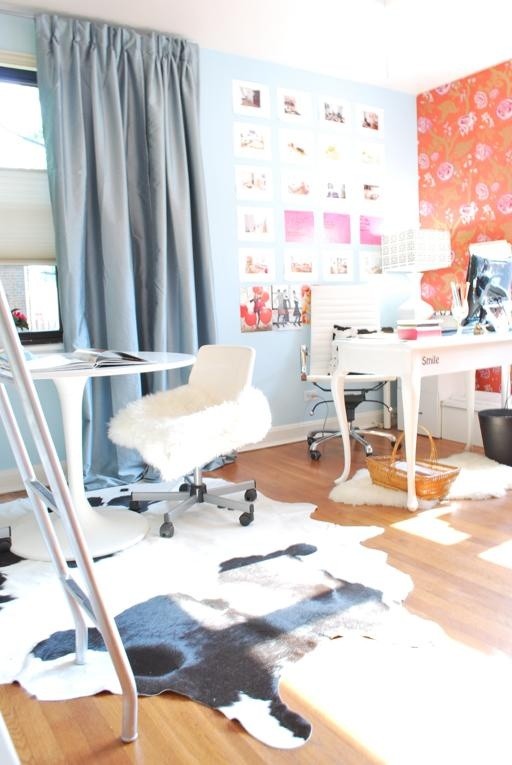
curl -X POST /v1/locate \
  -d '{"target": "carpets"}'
[328,452,511,506]
[0,475,416,751]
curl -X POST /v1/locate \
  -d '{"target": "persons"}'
[249,294,266,326]
[274,288,303,327]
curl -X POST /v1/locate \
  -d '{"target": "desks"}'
[0,351,195,561]
[329,331,512,513]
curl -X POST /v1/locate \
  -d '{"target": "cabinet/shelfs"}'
[396,320,465,438]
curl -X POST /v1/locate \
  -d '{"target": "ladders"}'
[0,278,140,743]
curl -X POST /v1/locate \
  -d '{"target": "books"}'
[27,348,150,372]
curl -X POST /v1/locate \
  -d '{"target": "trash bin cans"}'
[478,409,512,468]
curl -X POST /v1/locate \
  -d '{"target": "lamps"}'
[381,228,453,320]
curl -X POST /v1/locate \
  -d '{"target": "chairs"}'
[105,344,258,536]
[300,283,402,461]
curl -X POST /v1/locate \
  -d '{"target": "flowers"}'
[10,306,30,334]
